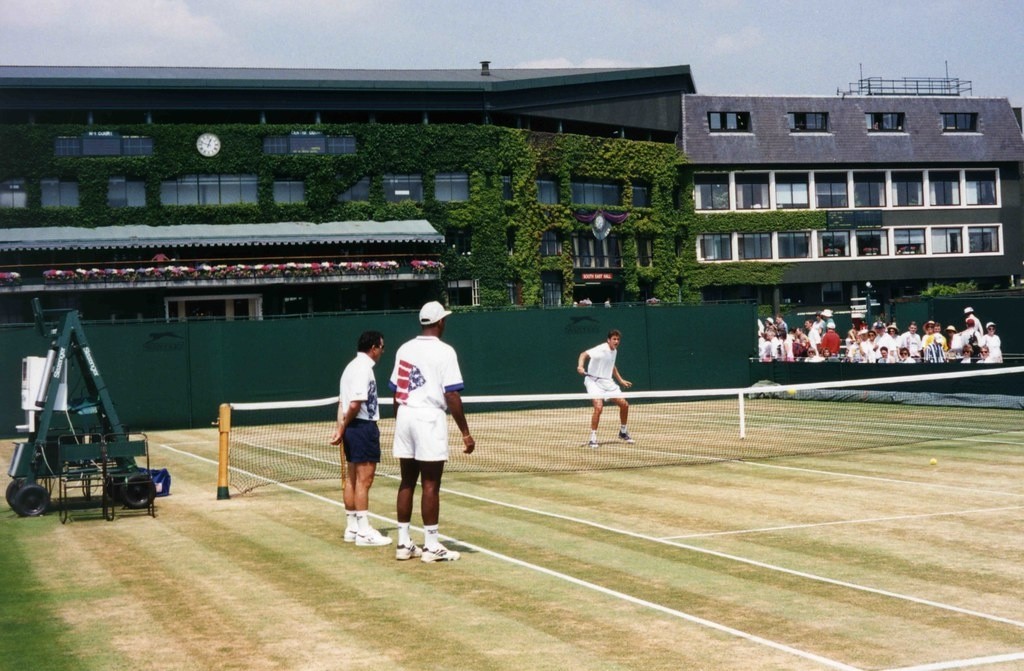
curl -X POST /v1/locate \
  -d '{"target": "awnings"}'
[0,219,446,251]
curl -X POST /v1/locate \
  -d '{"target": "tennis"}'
[788,389,796,394]
[930,458,937,465]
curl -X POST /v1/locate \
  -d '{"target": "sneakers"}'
[421,546,461,564]
[619,430,635,443]
[396,543,422,560]
[589,435,599,448]
[357,529,393,547]
[344,526,358,541]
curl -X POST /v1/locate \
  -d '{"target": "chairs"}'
[54,433,157,524]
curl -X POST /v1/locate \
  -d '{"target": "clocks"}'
[196,132,223,158]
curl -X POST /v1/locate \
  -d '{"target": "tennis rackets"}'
[584,372,618,391]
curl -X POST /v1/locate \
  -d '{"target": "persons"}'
[389,301,475,562]
[576,330,635,448]
[758,307,1003,363]
[329,331,393,547]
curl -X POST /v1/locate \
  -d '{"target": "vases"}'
[901,251,916,254]
[827,254,839,257]
[865,252,878,255]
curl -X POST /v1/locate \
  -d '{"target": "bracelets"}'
[463,435,470,439]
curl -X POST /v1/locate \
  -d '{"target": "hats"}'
[944,325,958,333]
[766,317,774,324]
[820,308,834,317]
[985,322,996,329]
[827,322,836,329]
[964,306,974,314]
[923,321,935,333]
[967,319,975,326]
[419,300,453,325]
[887,324,899,332]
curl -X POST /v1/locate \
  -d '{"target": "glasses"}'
[988,328,995,331]
[374,344,386,350]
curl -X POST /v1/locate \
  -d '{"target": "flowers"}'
[0,259,446,281]
[899,246,917,251]
[862,247,878,253]
[825,248,840,255]
